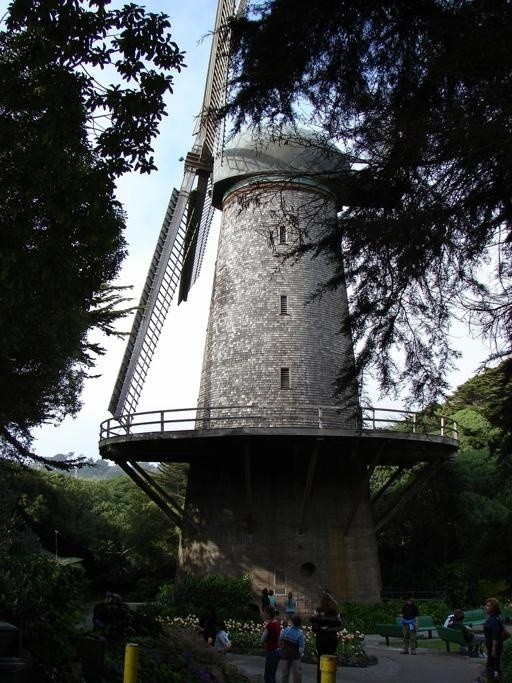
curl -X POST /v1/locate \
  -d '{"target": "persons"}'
[443,609,466,654]
[196,588,343,683]
[447,609,488,658]
[399,596,419,655]
[483,598,504,683]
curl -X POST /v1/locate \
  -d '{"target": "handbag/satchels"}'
[491,617,511,642]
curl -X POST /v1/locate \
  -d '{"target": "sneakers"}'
[399,649,409,654]
[410,648,417,655]
[458,646,488,658]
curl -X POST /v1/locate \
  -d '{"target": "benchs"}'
[396,615,436,638]
[462,608,487,631]
[376,622,417,647]
[435,624,472,653]
